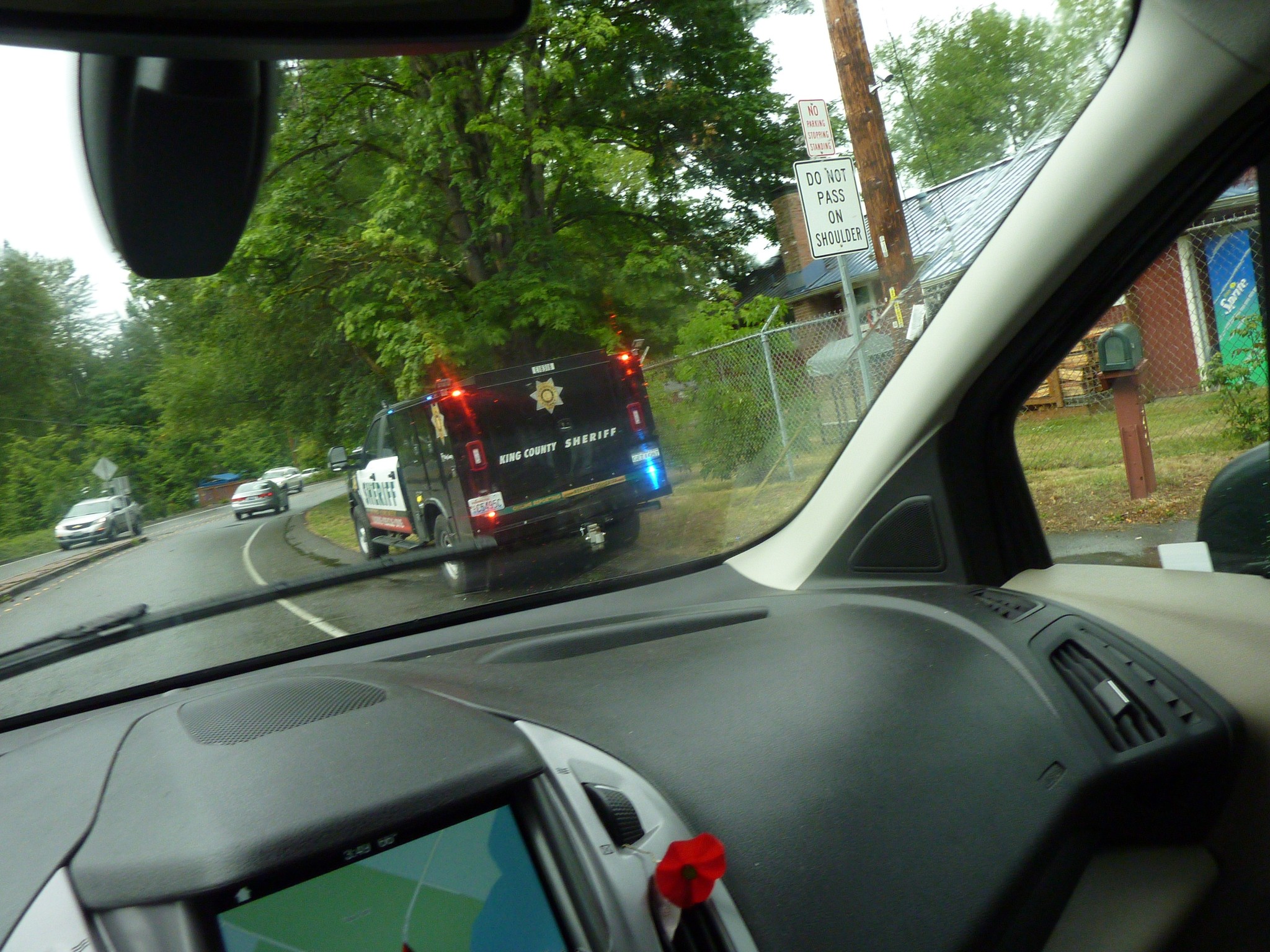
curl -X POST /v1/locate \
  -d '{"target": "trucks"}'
[327,329,675,594]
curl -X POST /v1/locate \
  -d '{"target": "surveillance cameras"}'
[875,68,894,83]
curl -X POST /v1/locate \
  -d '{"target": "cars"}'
[258,467,304,493]
[301,468,319,479]
[54,494,144,550]
[230,479,289,520]
